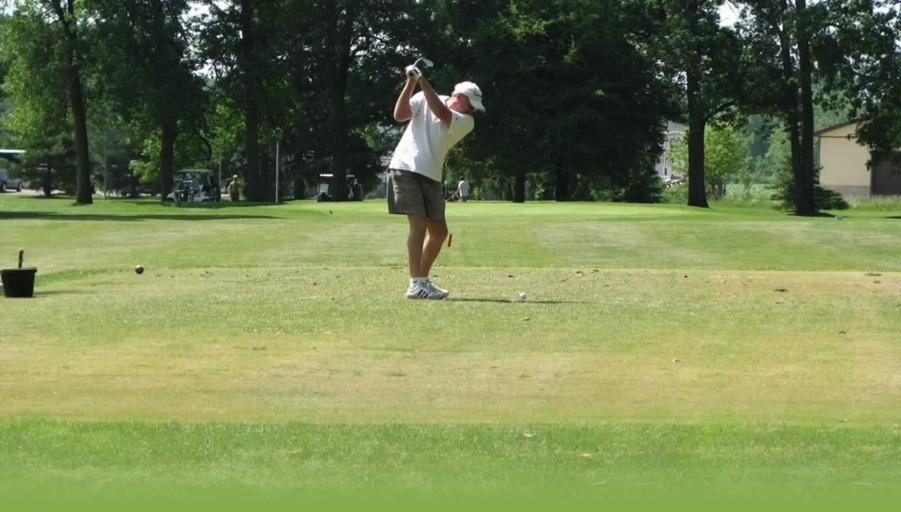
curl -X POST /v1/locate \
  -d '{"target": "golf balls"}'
[135,265,144,273]
[519,292,526,300]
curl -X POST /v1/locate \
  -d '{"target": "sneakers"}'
[425,278,449,300]
[406,280,446,299]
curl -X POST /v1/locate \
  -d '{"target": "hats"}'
[454,81,486,114]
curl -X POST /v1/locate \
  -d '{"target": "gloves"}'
[403,65,421,82]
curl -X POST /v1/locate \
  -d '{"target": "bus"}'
[280,173,355,199]
[0,148,56,192]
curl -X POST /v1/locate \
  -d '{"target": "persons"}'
[348,177,363,200]
[457,176,470,200]
[386,65,486,300]
[169,172,240,201]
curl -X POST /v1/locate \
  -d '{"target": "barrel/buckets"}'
[1,268,37,296]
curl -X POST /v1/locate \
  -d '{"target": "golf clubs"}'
[408,57,433,75]
